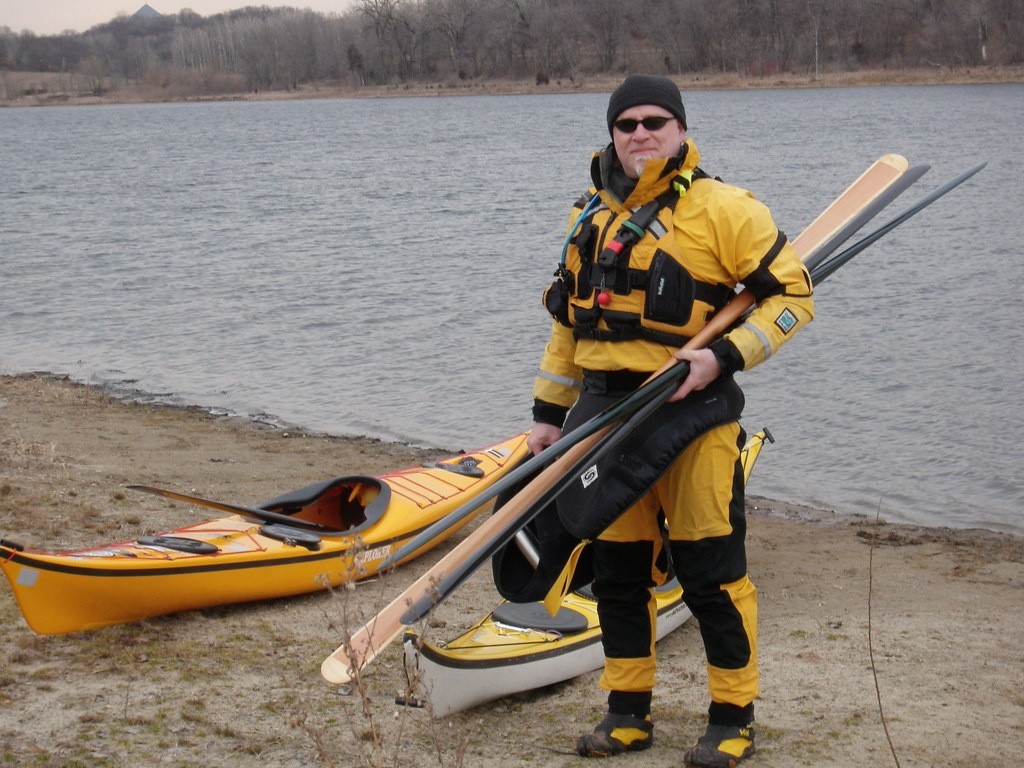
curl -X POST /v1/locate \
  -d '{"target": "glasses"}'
[613,115,679,134]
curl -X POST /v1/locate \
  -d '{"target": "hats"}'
[606,73,688,140]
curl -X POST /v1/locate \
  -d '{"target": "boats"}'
[2,418,541,637]
[402,428,779,723]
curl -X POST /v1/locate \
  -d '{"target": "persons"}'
[529,73,815,768]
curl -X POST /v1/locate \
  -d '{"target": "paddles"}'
[318,152,988,685]
[124,480,342,532]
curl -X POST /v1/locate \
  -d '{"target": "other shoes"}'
[576,712,654,758]
[683,713,756,767]
